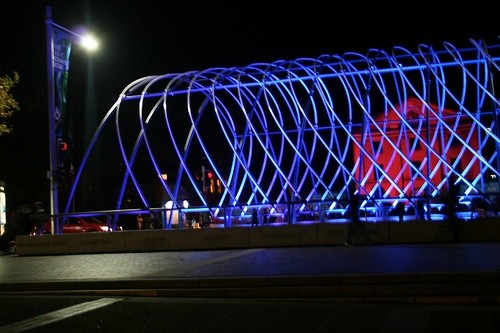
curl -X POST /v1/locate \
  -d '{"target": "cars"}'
[31,216,123,235]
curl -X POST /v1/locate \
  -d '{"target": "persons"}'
[135,200,426,229]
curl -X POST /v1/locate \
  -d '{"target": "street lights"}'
[45,18,96,239]
